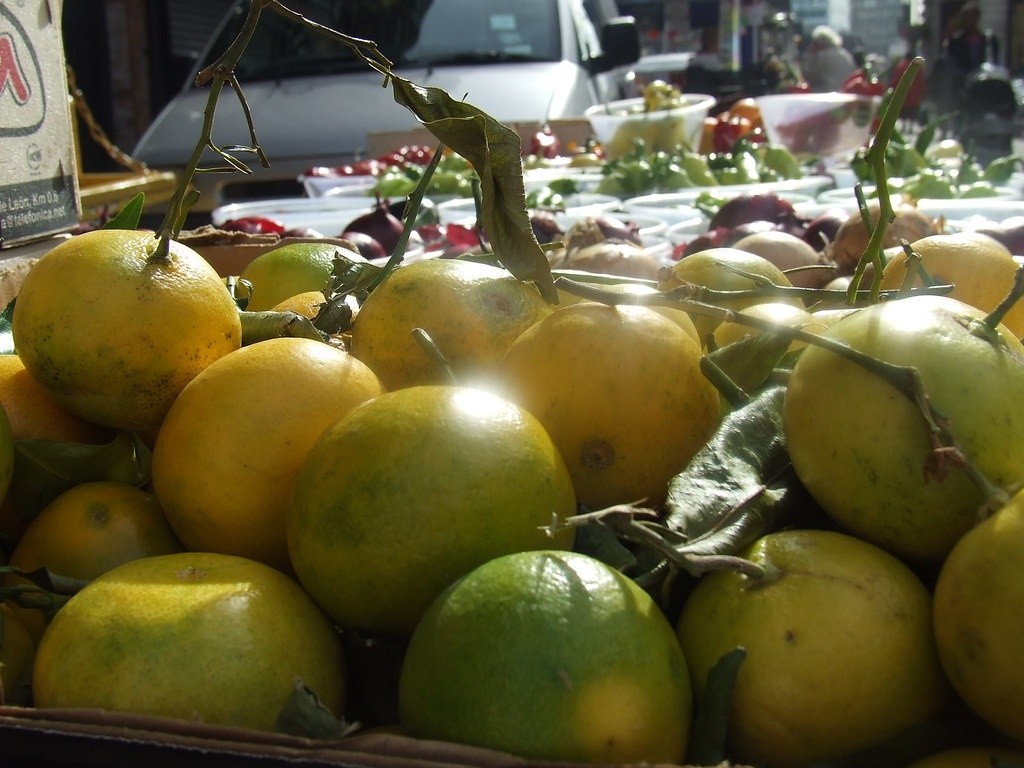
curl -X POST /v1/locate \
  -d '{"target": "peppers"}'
[301,55,1024,203]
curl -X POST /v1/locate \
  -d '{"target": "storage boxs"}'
[370,119,586,170]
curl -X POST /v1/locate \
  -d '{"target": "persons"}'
[683,25,720,98]
[853,50,923,120]
[966,62,1015,166]
[947,1,985,68]
[805,25,857,93]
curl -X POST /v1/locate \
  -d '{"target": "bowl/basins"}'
[583,93,717,159]
[755,92,883,159]
[209,152,1024,236]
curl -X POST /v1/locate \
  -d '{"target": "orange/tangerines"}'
[0,234,1024,768]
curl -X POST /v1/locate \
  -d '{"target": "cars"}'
[129,0,644,179]
